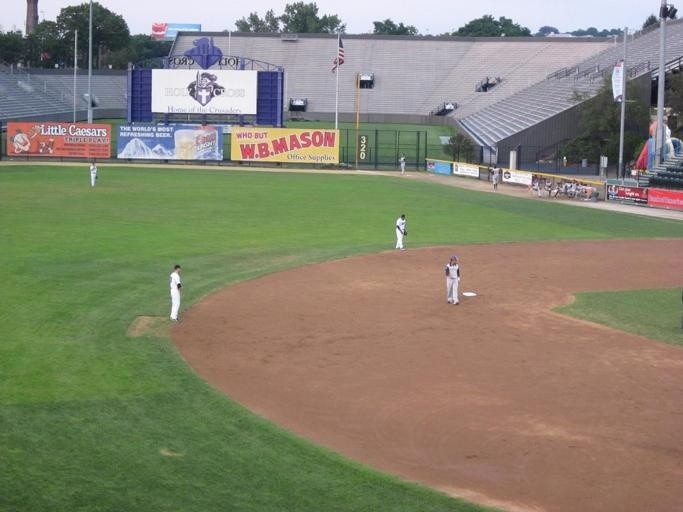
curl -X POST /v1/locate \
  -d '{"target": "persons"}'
[444,255,461,305]
[398,151,406,174]
[491,164,503,192]
[90,161,98,187]
[528,175,597,201]
[169,264,183,321]
[394,213,408,251]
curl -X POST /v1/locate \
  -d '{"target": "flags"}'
[610,59,626,101]
[329,40,345,74]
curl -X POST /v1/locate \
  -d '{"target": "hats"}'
[174,265,184,269]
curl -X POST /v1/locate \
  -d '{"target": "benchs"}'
[0,21,682,192]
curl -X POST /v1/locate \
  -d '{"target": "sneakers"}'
[400,248,406,251]
[447,298,460,305]
[169,317,181,321]
